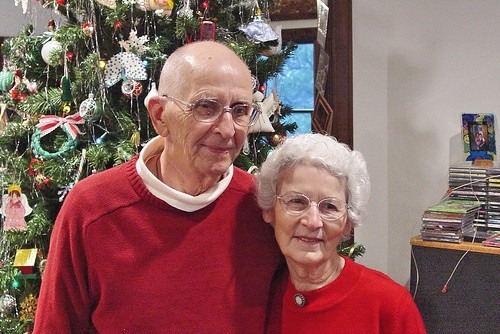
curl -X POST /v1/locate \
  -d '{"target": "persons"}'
[255,134,425,334]
[32,40,279,334]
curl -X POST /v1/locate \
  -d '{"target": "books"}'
[420,113,500,245]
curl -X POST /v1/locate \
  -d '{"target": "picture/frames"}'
[462,113,497,160]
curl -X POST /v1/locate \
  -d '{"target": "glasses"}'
[162,95,263,127]
[275,191,351,222]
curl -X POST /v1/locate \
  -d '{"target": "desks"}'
[410,235,500,334]
